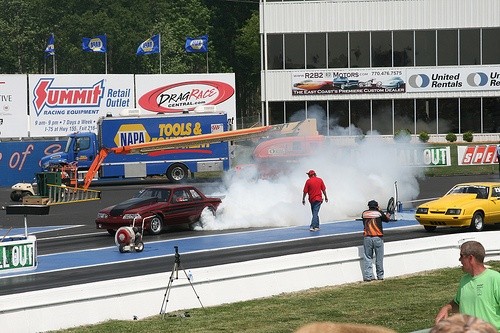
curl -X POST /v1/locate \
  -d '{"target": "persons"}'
[296,320,394,333]
[434,240,500,333]
[428,312,497,333]
[302,170,328,231]
[362,200,390,282]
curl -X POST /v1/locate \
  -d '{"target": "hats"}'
[306,170,315,174]
[368,200,378,206]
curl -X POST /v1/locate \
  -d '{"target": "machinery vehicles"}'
[9,122,281,203]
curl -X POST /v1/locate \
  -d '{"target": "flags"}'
[82,36,107,52]
[136,35,160,55]
[185,35,208,52]
[45,36,55,57]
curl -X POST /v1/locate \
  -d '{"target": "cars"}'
[292,75,384,95]
[95,183,222,237]
[415,182,500,233]
[380,75,406,91]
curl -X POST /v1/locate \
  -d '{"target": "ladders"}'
[46,183,101,205]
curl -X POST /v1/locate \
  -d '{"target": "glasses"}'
[460,253,472,258]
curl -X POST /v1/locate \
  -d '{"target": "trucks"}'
[37,111,234,185]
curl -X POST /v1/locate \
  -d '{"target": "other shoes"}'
[315,228,320,230]
[310,228,314,231]
